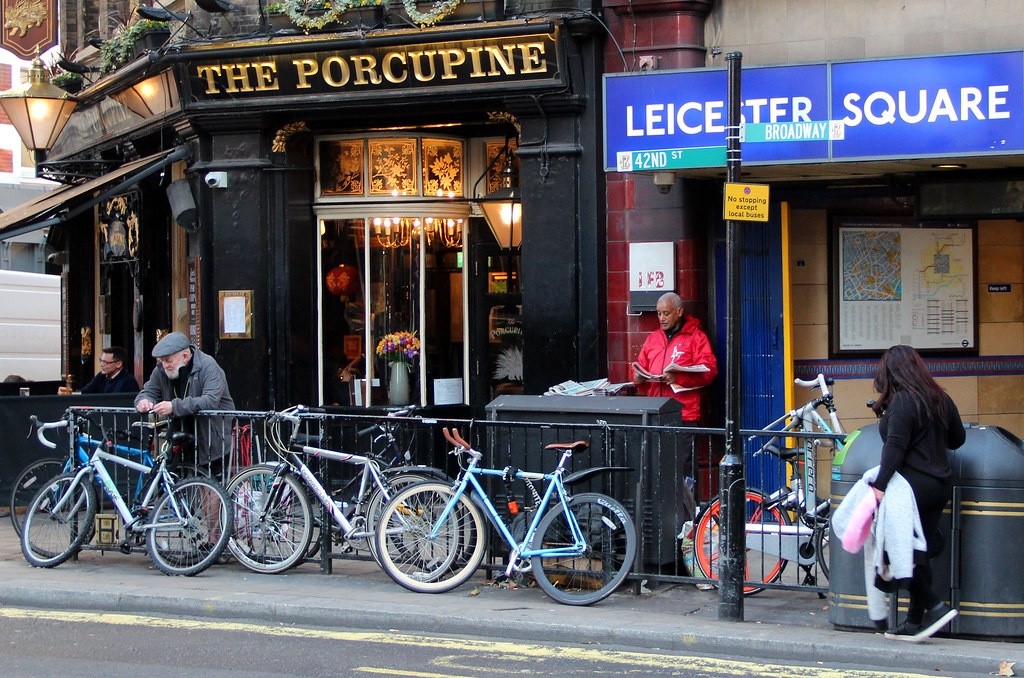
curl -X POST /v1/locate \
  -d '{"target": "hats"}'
[152,332,189,358]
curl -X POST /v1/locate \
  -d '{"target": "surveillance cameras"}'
[47,252,58,265]
[653,172,675,194]
[204,173,220,187]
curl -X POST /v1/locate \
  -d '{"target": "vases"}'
[269,5,380,34]
[391,363,408,405]
[387,0,504,26]
[122,28,171,64]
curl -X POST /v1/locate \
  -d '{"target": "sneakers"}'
[884,620,921,641]
[914,602,958,640]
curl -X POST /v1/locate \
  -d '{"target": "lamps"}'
[0,44,112,185]
[108,68,179,118]
[86,38,105,48]
[374,189,463,249]
[465,125,522,256]
[56,59,105,76]
[195,0,231,13]
[136,7,192,22]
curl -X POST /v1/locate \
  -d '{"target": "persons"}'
[133,333,236,560]
[873,345,965,641]
[632,292,717,506]
[323,353,365,405]
[4,375,25,382]
[78,347,140,392]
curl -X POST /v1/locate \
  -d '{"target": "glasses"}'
[99,358,118,365]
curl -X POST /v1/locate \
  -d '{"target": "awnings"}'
[0,145,191,241]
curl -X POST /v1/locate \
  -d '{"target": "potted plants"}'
[52,73,83,93]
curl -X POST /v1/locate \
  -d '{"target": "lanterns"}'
[326,265,360,302]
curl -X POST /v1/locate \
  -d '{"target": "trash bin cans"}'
[826,423,1024,644]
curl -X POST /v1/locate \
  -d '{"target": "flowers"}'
[260,0,461,30]
[98,20,170,73]
[376,330,420,371]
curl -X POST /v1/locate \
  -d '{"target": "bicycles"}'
[371,425,639,609]
[694,373,884,601]
[7,403,476,584]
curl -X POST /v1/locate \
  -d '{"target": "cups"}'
[20,387,30,397]
